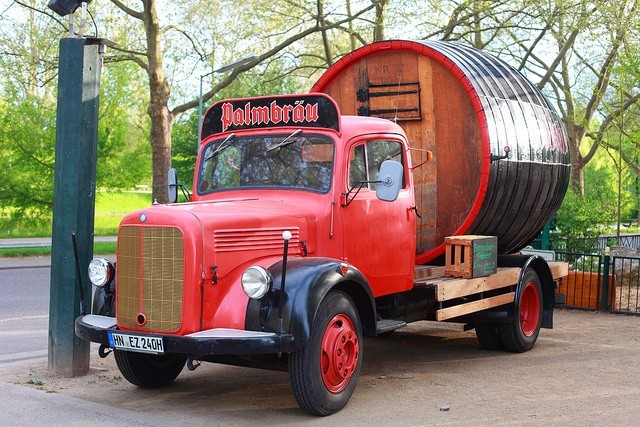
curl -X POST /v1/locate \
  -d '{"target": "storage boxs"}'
[444,235,498,277]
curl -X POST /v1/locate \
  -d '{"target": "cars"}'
[71,39,571,417]
